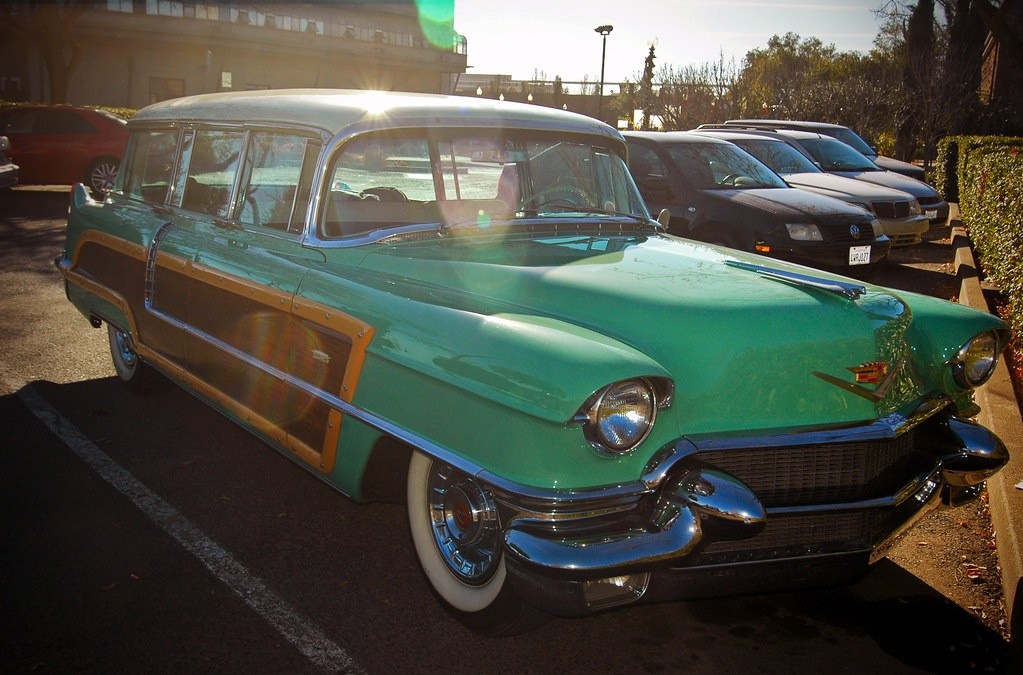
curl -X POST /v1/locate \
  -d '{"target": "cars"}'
[724,117,932,186]
[492,132,889,282]
[0,102,175,201]
[57,85,1013,635]
[750,131,953,241]
[690,123,929,251]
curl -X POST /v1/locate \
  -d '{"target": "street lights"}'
[594,24,614,121]
